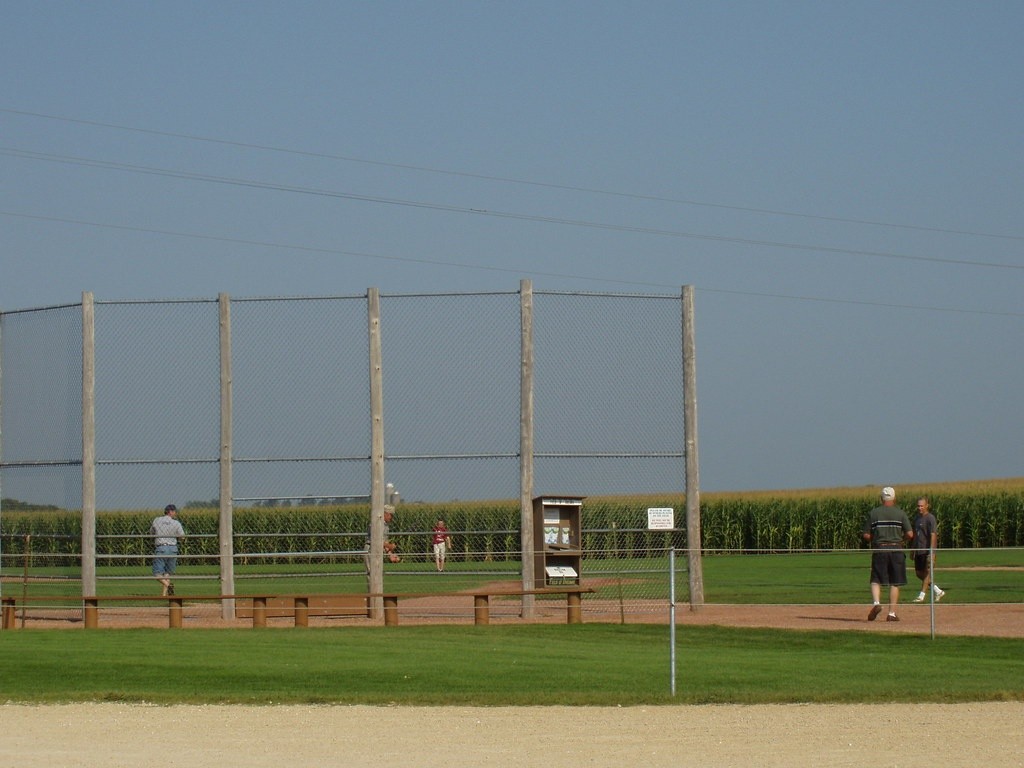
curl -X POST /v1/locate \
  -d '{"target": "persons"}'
[910,496,946,603]
[149,504,185,596]
[363,504,400,582]
[863,486,913,621]
[431,519,451,572]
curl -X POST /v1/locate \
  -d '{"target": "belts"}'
[881,543,901,546]
[157,544,171,546]
[366,542,370,545]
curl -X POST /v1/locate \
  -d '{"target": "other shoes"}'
[437,569,440,572]
[168,583,175,596]
[868,604,881,621]
[934,590,946,602]
[441,569,443,572]
[911,597,925,604]
[887,612,900,620]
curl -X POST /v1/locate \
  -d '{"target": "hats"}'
[165,505,176,510]
[881,487,895,501]
[384,504,395,513]
